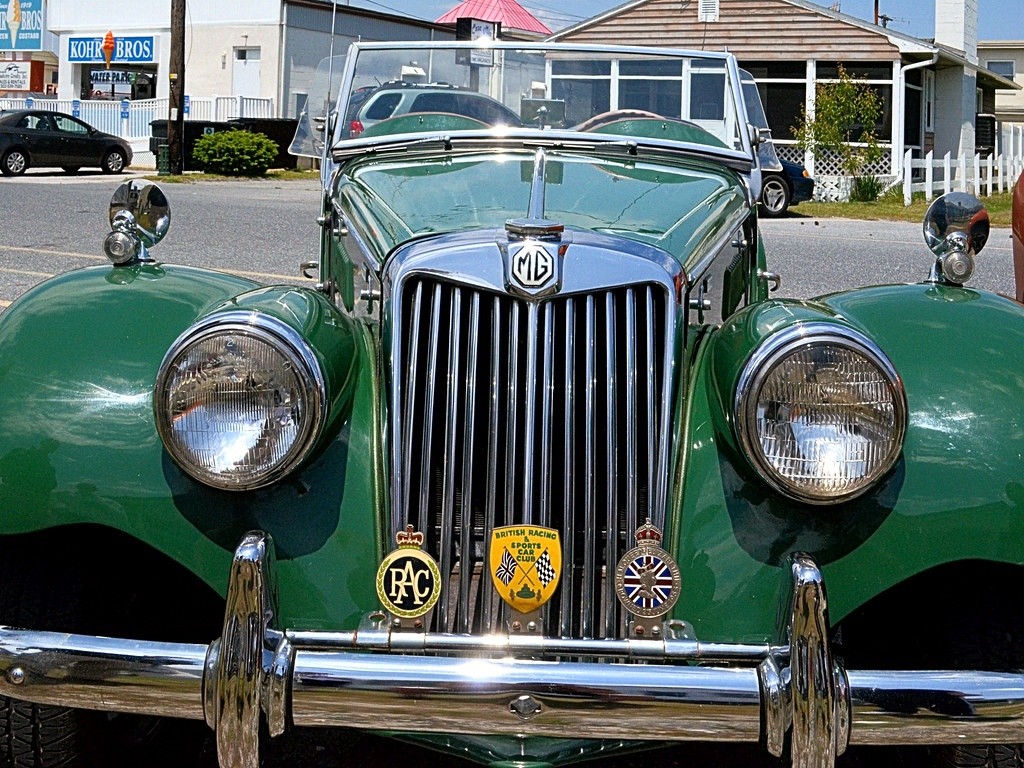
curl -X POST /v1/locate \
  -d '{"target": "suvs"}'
[322,79,528,135]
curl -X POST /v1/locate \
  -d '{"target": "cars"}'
[757,148,815,218]
[0,108,134,176]
[1012,169,1023,304]
[0,42,1023,767]
